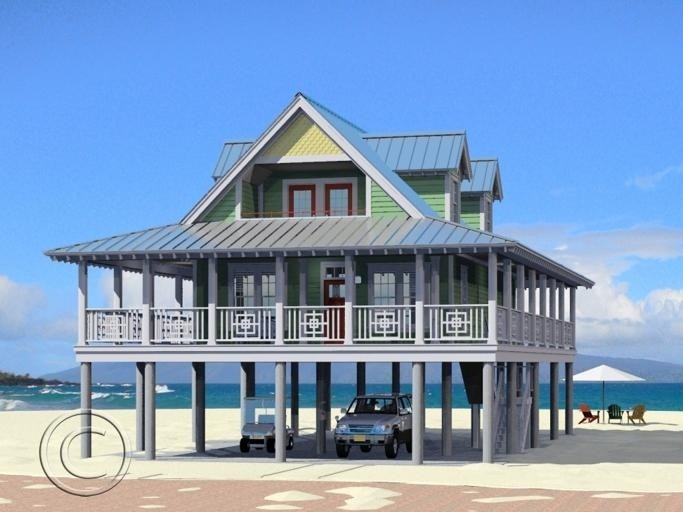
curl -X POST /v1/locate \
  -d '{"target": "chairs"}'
[577,403,646,425]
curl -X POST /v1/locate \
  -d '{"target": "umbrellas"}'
[559,362,645,422]
